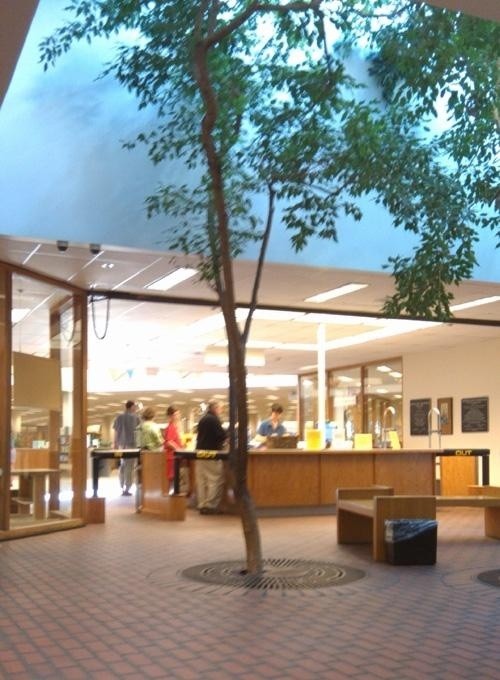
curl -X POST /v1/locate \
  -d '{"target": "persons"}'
[134,407,165,449]
[194,401,228,517]
[161,405,187,492]
[112,399,141,497]
[254,402,288,444]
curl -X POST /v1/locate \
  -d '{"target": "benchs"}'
[336,484,500,566]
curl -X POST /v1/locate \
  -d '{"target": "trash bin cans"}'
[384,518,438,565]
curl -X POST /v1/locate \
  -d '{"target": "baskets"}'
[265,433,300,449]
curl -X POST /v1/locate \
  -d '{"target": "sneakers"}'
[122,491,132,496]
[200,507,223,515]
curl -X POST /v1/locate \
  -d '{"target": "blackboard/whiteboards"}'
[461,397,488,431]
[410,399,431,435]
